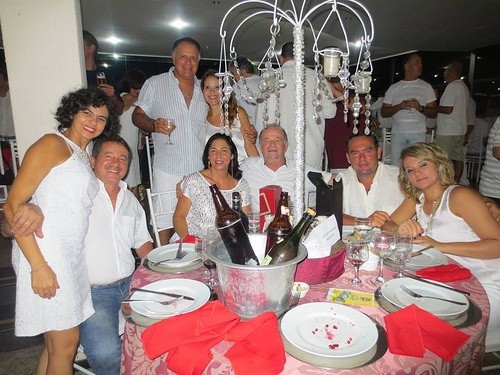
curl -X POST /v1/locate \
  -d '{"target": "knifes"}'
[178,237,183,254]
[411,245,435,257]
[131,287,195,301]
[402,273,470,295]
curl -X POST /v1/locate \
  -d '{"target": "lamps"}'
[214,0,375,227]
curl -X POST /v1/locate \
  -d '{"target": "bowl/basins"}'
[291,282,310,298]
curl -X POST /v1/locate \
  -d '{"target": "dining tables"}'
[121,228,500,375]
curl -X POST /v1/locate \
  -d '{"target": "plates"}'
[146,242,201,265]
[143,259,205,274]
[281,302,379,358]
[121,290,162,327]
[387,243,448,268]
[380,277,469,320]
[128,278,211,320]
[278,315,377,369]
[373,285,468,326]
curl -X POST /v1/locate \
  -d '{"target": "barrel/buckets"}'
[203,233,309,319]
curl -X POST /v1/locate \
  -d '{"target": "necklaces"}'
[425,197,437,204]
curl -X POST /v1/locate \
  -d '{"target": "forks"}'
[119,296,184,306]
[400,285,467,305]
[276,290,301,320]
[154,251,188,266]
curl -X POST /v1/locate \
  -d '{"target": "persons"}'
[83,30,154,203]
[228,41,392,214]
[381,142,500,331]
[462,80,500,209]
[201,69,260,162]
[132,38,257,247]
[176,124,337,232]
[381,54,438,167]
[436,64,469,183]
[0,62,20,185]
[334,131,500,231]
[2,88,121,375]
[1,135,154,375]
[172,133,252,242]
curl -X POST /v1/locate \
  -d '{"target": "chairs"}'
[466,127,485,181]
[381,128,435,167]
[145,189,177,245]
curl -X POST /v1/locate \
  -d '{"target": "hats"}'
[443,61,464,77]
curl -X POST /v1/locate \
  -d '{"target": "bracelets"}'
[420,106,424,113]
[31,263,47,272]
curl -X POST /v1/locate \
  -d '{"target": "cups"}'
[354,216,372,244]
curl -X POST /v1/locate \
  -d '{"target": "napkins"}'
[384,304,472,362]
[141,301,286,375]
[417,264,471,283]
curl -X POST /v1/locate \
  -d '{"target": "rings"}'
[44,291,49,294]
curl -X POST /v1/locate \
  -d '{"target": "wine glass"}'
[393,232,413,279]
[346,240,369,287]
[369,230,397,287]
[163,118,175,145]
[407,96,415,115]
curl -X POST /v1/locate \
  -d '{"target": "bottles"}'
[208,184,258,266]
[230,191,249,234]
[264,190,293,257]
[261,207,317,266]
